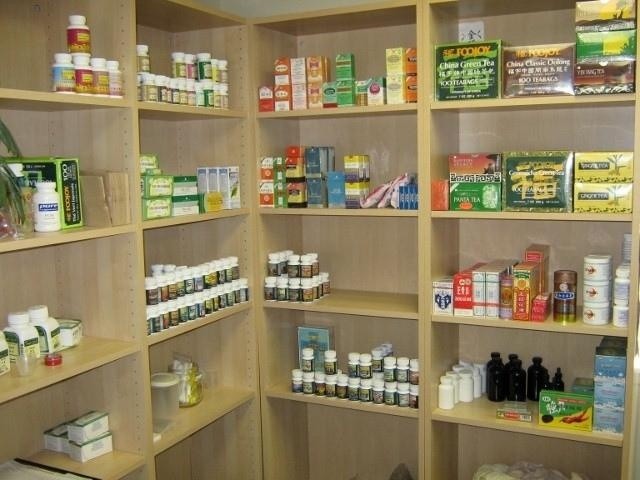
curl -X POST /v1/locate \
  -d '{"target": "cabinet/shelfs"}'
[0,0,264,480]
[248,1,639,480]
